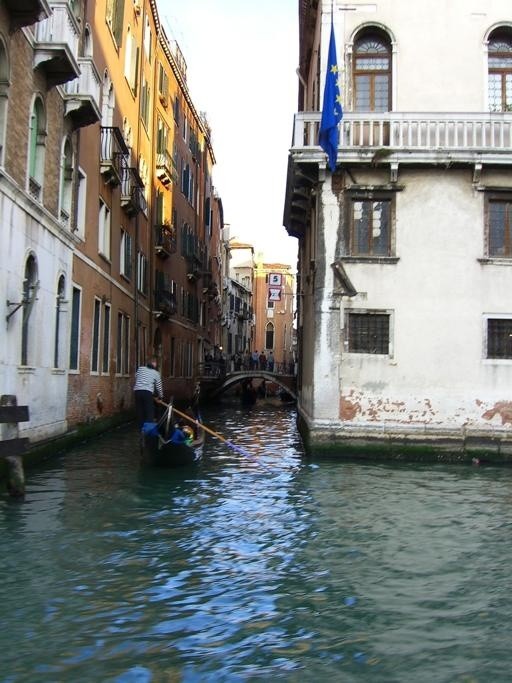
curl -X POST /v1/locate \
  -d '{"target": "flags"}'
[318,20,343,172]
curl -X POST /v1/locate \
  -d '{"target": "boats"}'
[138,404,206,465]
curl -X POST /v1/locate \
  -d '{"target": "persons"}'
[134,361,163,422]
[204,350,274,375]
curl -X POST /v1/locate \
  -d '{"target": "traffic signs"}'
[269,272,282,286]
